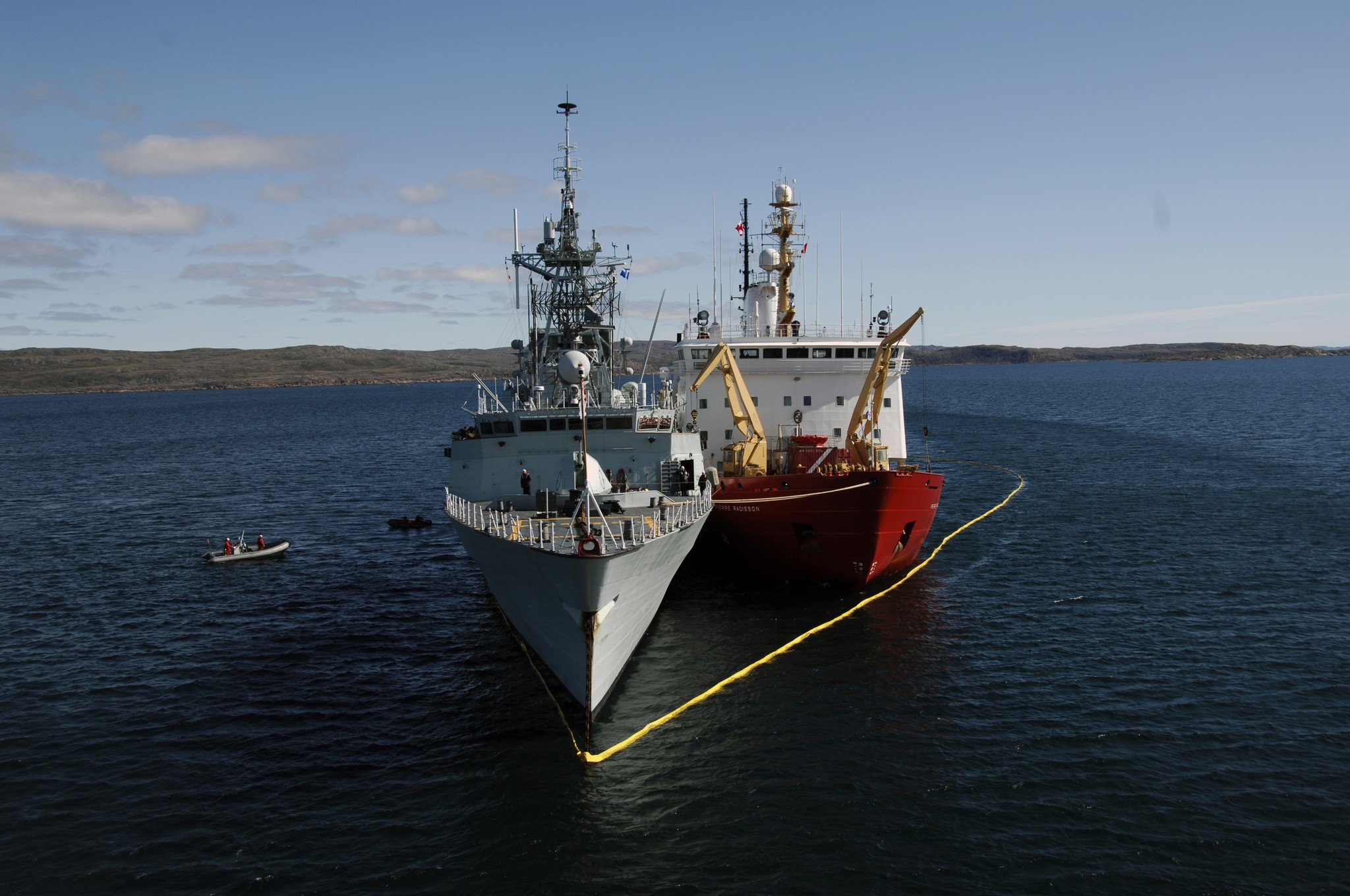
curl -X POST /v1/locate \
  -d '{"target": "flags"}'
[735,219,744,236]
[578,378,587,420]
[620,267,630,280]
[801,243,808,254]
[866,406,873,421]
[509,276,511,282]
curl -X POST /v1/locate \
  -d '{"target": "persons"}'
[225,538,233,555]
[674,466,690,496]
[520,469,532,495]
[401,516,412,524]
[832,466,837,475]
[866,460,872,471]
[414,514,424,524]
[879,464,886,471]
[698,471,708,498]
[257,535,265,550]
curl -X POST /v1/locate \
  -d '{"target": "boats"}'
[790,435,828,445]
[675,166,943,599]
[200,529,291,564]
[387,515,432,529]
[442,91,715,729]
